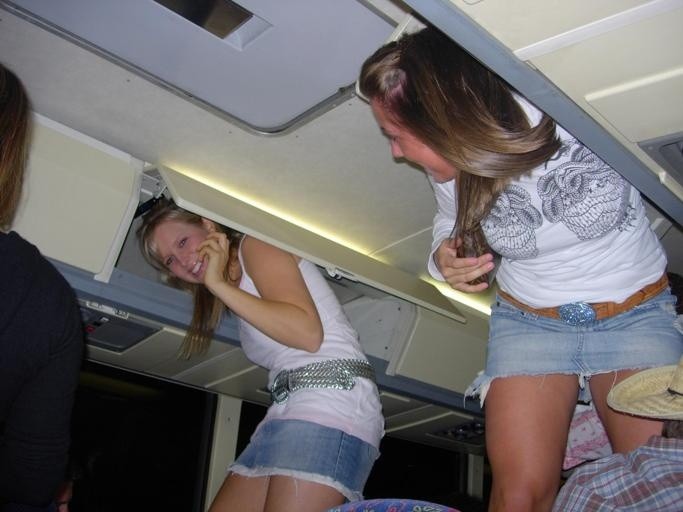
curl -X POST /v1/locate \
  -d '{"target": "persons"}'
[138,199,386,511]
[0,66,86,512]
[359,28,683,512]
[550,355,683,512]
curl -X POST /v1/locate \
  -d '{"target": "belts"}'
[493,271,670,326]
[270,358,377,404]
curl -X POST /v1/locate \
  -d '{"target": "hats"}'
[606,353,682,421]
